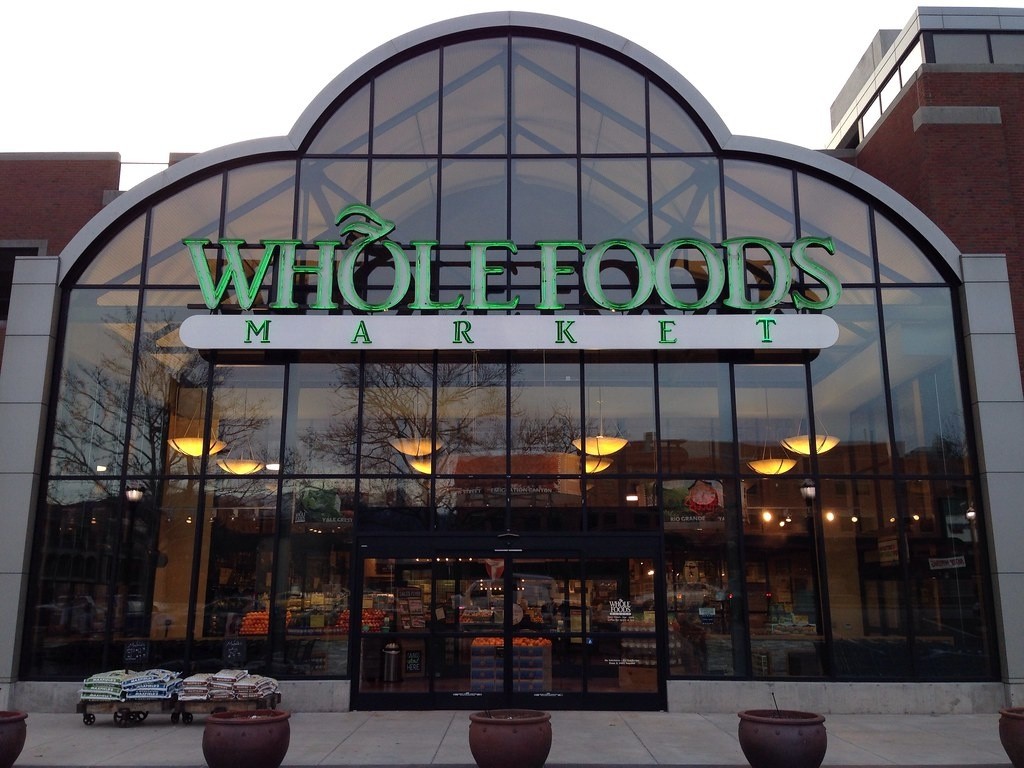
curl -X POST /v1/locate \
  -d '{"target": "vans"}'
[461,579,509,616]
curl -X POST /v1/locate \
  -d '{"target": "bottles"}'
[620,635,683,666]
[557,620,564,641]
[526,608,538,619]
[489,607,496,622]
[620,616,680,632]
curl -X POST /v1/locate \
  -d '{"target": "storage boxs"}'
[471,637,552,694]
[766,604,816,635]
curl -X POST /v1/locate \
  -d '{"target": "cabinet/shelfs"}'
[621,630,682,668]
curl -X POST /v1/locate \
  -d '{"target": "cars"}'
[204,598,267,637]
[633,580,717,612]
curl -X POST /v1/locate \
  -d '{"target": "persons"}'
[541,598,558,629]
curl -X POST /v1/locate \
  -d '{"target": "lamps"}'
[573,387,629,474]
[390,386,444,474]
[167,387,263,473]
[745,386,840,475]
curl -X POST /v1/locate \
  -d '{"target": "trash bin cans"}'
[383,642,401,682]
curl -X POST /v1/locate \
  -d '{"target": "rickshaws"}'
[75,695,283,724]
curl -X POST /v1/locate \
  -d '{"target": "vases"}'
[999,708,1024,768]
[0,710,29,768]
[736,709,828,768]
[469,709,552,768]
[201,712,293,768]
